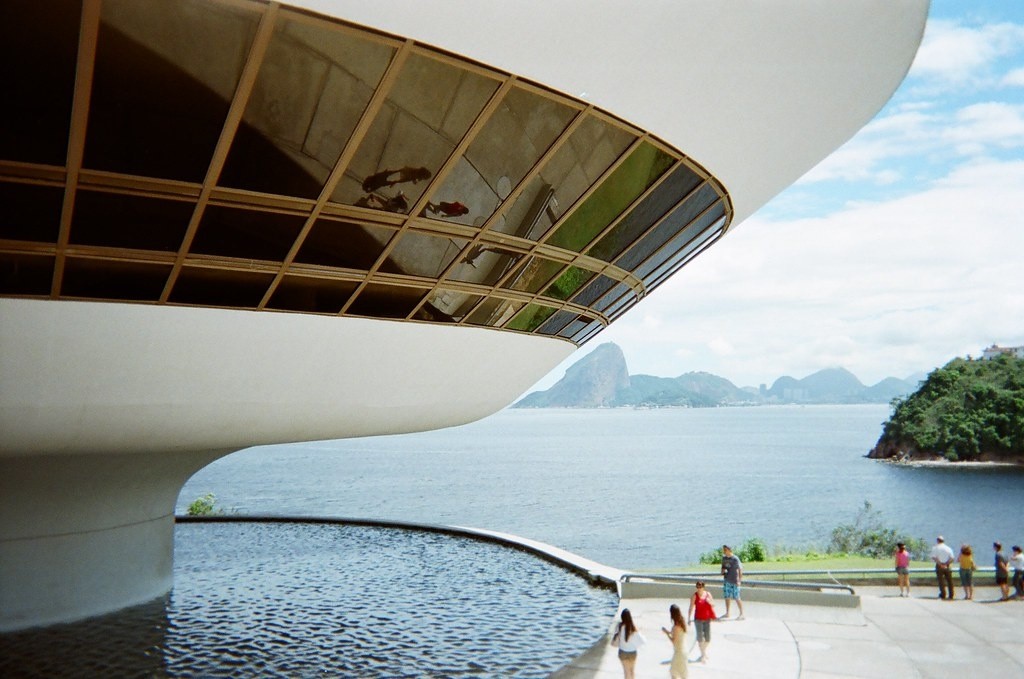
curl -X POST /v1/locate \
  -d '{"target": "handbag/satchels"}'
[710,606,717,620]
[611,623,621,647]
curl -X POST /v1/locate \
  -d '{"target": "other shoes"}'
[900,593,904,596]
[701,655,709,665]
[721,615,729,617]
[907,593,911,597]
[736,616,745,620]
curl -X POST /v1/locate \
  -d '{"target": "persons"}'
[956,545,975,600]
[660,603,690,679]
[992,542,1011,601]
[720,544,746,621]
[354,167,520,266]
[930,536,954,600]
[687,581,715,663]
[1011,545,1024,601]
[611,609,645,679]
[894,541,911,597]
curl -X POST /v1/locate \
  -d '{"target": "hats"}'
[897,541,906,546]
